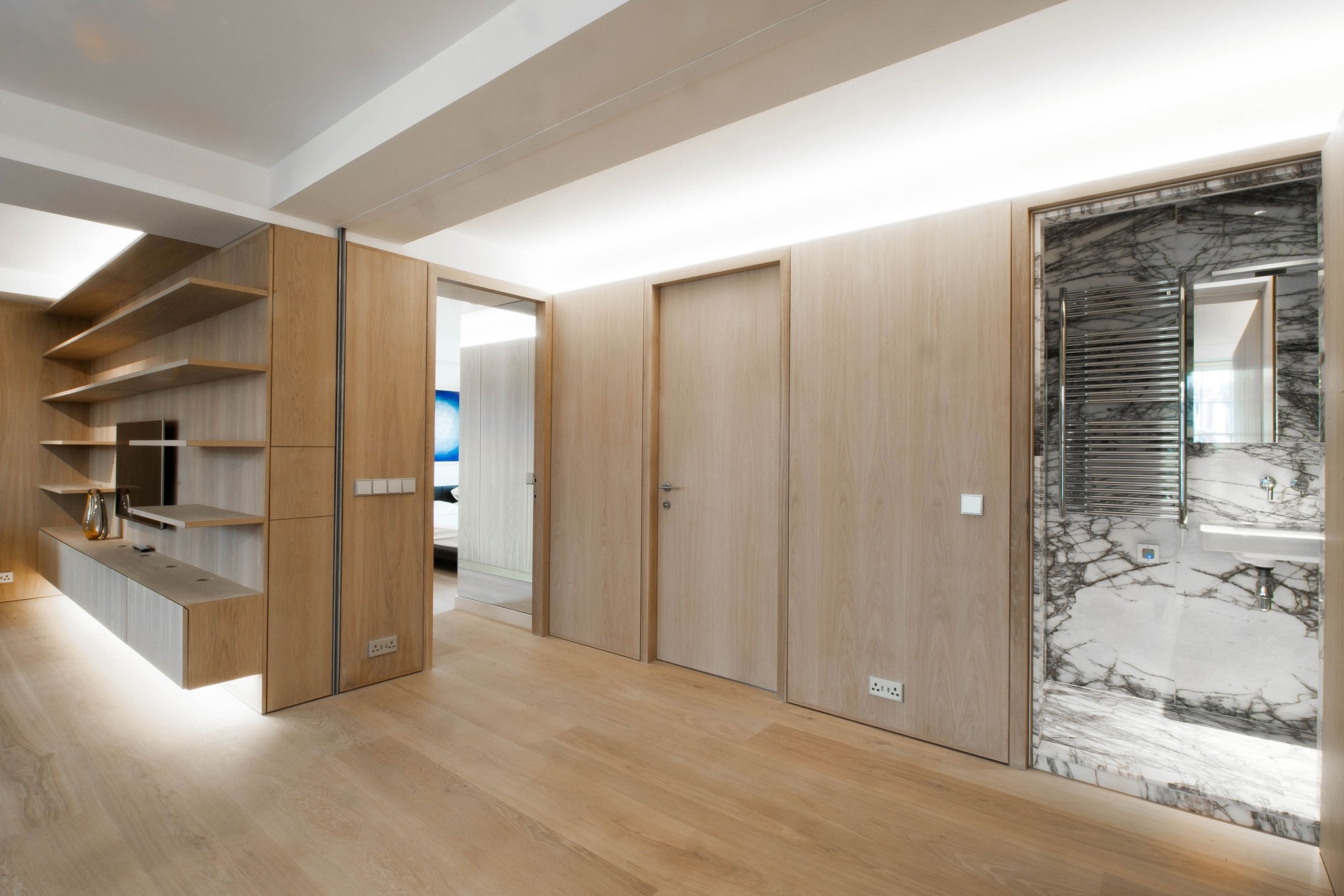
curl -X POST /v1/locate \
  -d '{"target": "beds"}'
[433,484,459,573]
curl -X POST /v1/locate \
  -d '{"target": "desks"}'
[868,675,904,704]
[367,635,398,659]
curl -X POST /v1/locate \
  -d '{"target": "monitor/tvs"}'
[114,419,170,530]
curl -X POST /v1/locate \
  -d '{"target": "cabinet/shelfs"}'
[38,231,266,694]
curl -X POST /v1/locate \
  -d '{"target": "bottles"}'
[116,487,132,519]
[82,488,108,541]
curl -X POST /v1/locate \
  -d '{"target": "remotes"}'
[133,545,151,552]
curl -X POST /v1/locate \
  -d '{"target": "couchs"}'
[451,486,458,502]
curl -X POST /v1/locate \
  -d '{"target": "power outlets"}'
[0,572,13,584]
